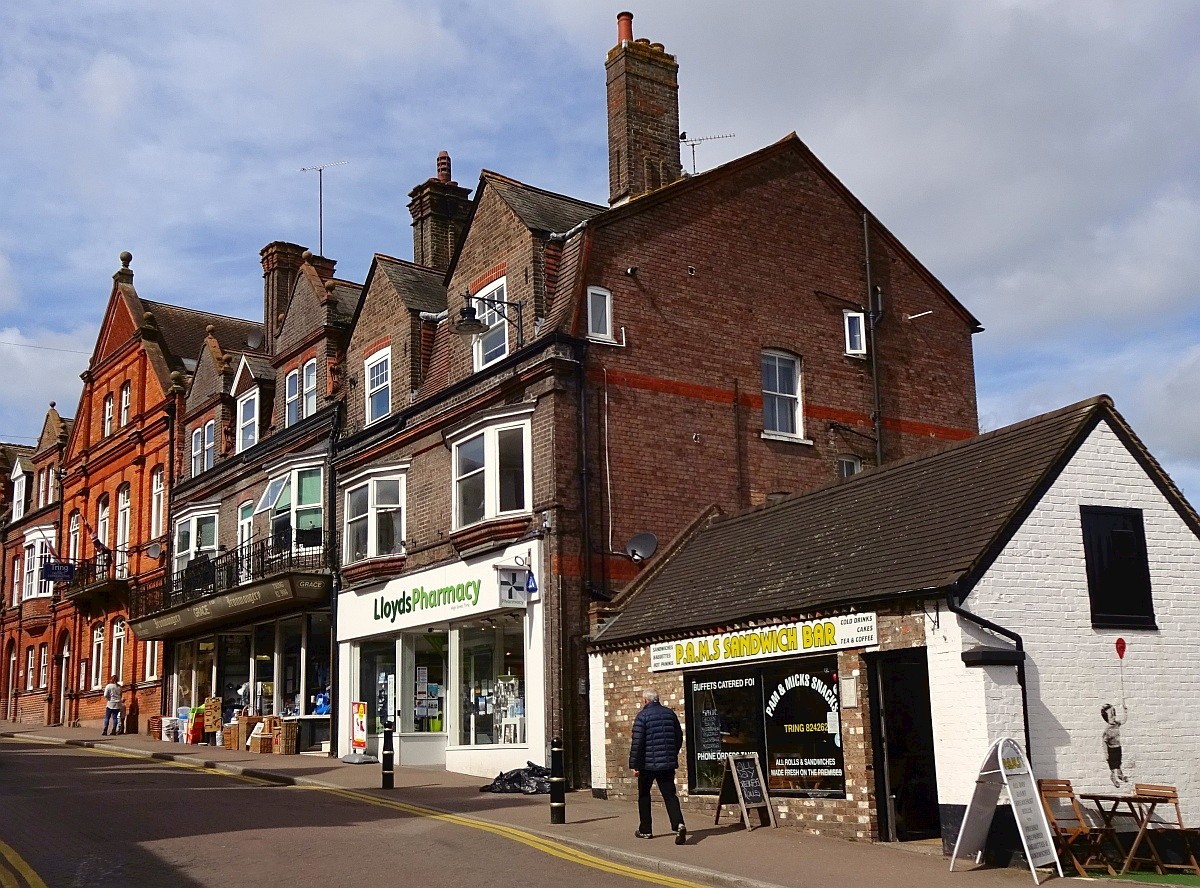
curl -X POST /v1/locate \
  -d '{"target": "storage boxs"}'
[161,696,299,755]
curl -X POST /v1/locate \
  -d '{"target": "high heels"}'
[110,731,117,736]
[102,731,107,736]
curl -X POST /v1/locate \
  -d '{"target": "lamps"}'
[510,612,520,624]
[486,618,497,629]
[448,286,522,348]
[825,421,877,446]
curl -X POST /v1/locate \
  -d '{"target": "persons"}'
[102,675,122,736]
[629,689,687,845]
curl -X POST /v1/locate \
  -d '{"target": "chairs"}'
[1038,779,1117,878]
[1136,784,1200,876]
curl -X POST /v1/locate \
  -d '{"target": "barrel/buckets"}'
[177,707,192,719]
[150,716,162,739]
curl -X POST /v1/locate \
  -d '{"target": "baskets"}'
[239,705,261,724]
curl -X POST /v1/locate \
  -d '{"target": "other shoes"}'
[635,830,652,838]
[117,730,124,735]
[675,825,687,845]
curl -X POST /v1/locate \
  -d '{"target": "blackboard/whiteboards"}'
[699,709,721,754]
[719,754,769,805]
[951,737,1056,866]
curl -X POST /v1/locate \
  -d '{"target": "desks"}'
[502,717,526,744]
[1080,794,1169,876]
